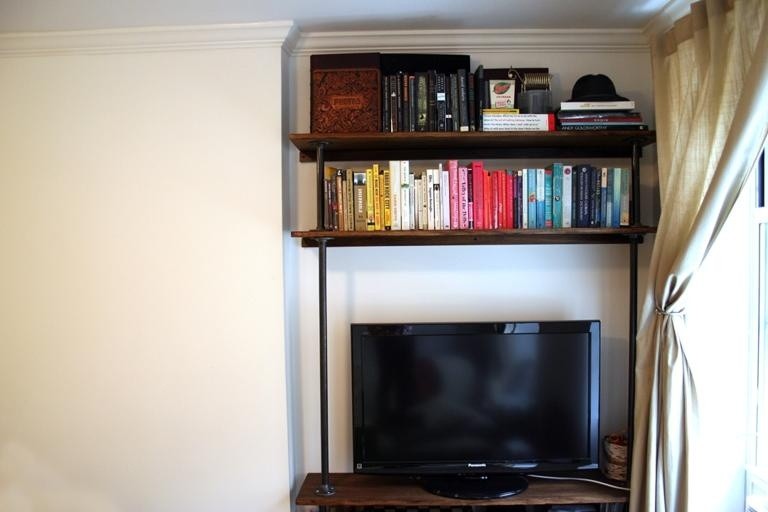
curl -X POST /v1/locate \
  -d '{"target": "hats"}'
[554,74,630,115]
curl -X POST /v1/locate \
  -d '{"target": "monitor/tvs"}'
[350,320,601,500]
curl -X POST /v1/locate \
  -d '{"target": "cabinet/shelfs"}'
[288,131,658,512]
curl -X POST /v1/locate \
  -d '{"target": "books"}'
[323,159,630,231]
[310,53,648,134]
